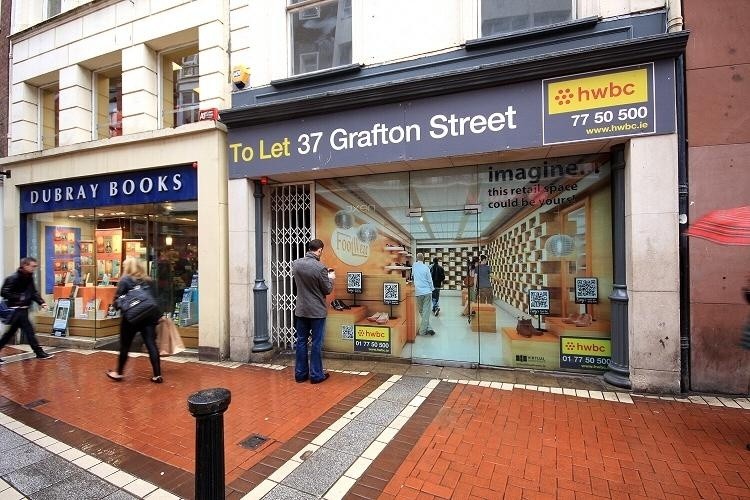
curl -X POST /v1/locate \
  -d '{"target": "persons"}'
[411,252,434,337]
[462,257,478,317]
[0,256,54,365]
[474,254,502,305]
[291,238,334,383]
[103,257,165,385]
[428,257,445,316]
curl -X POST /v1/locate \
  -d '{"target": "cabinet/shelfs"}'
[384,212,580,329]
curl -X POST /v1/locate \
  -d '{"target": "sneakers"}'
[331,300,343,312]
[427,330,435,335]
[324,373,330,379]
[367,312,381,322]
[563,313,592,327]
[432,307,440,317]
[36,350,55,360]
[376,313,390,324]
[338,299,352,310]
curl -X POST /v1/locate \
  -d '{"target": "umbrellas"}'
[679,202,750,248]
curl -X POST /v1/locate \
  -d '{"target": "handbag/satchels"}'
[154,316,187,356]
[464,277,474,287]
[114,275,158,324]
[0,300,17,325]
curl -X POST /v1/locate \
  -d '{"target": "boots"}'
[517,319,545,337]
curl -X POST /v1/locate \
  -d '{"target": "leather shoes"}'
[105,369,122,382]
[150,377,163,383]
